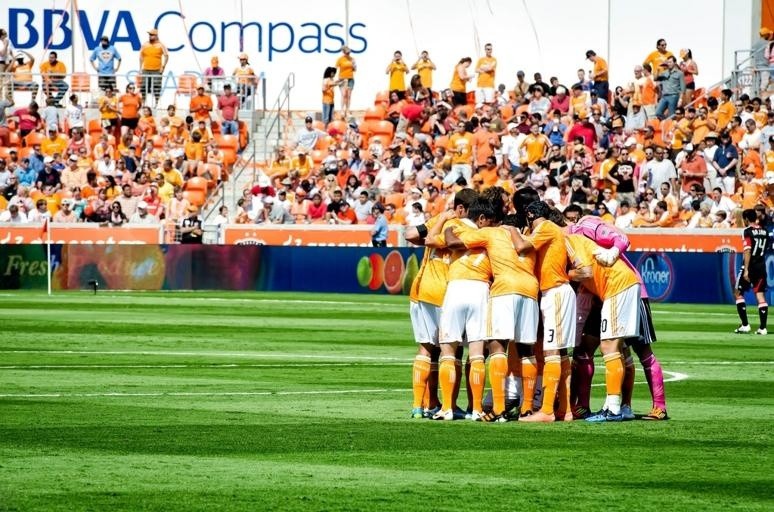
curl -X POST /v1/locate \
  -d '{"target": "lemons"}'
[383,250,404,292]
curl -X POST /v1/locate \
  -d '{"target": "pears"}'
[402,254,419,295]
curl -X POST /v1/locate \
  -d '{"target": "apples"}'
[358,256,372,287]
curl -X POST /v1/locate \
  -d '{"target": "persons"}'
[733,209,769,335]
[404,186,667,422]
[0,27,774,230]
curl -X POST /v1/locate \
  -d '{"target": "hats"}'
[62,198,71,204]
[187,206,198,212]
[148,28,158,35]
[238,52,248,59]
[684,143,695,152]
[372,202,386,210]
[624,137,637,147]
[69,155,79,161]
[49,125,57,131]
[264,151,309,205]
[305,116,312,123]
[411,187,423,195]
[42,157,56,164]
[389,132,426,150]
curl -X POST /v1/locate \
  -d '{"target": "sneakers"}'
[585,404,635,421]
[734,324,751,333]
[755,328,767,336]
[642,407,667,420]
[411,403,593,423]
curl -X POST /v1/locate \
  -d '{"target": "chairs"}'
[15,85,29,90]
[501,106,514,120]
[136,75,142,89]
[174,73,199,104]
[313,91,396,162]
[210,121,223,138]
[238,121,248,147]
[184,177,207,206]
[72,71,91,102]
[516,104,528,117]
[385,195,404,207]
[19,133,48,160]
[88,118,102,140]
[649,118,662,145]
[0,132,20,158]
[216,134,237,166]
